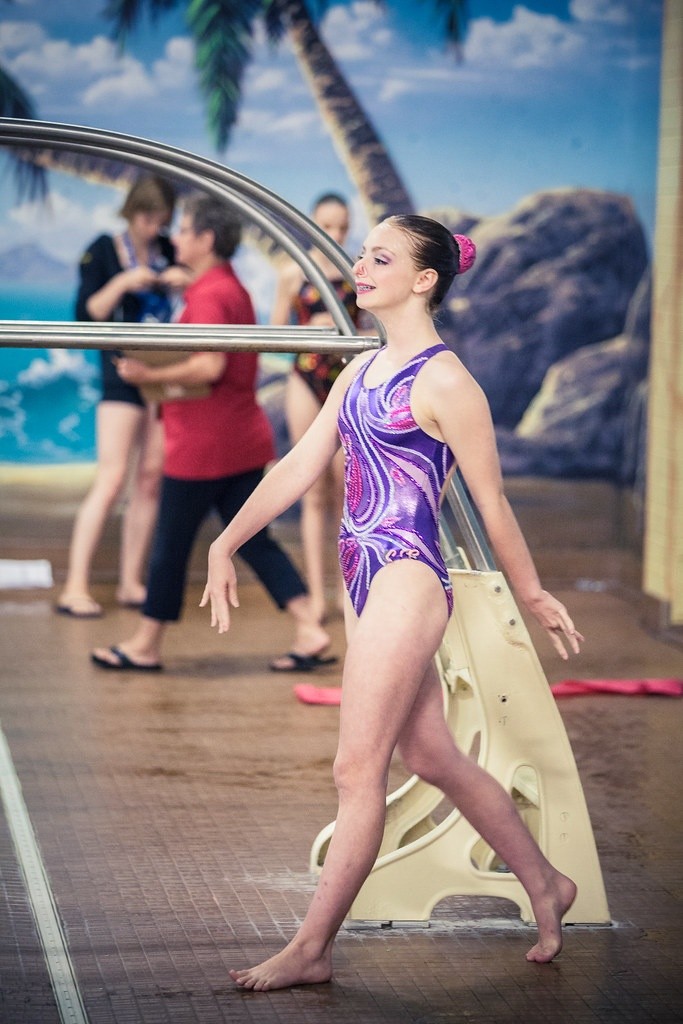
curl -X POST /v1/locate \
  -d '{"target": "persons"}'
[196,213,586,993]
[53,172,367,674]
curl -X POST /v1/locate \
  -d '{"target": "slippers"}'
[90,644,162,676]
[113,594,148,607]
[53,593,105,619]
[268,649,342,675]
